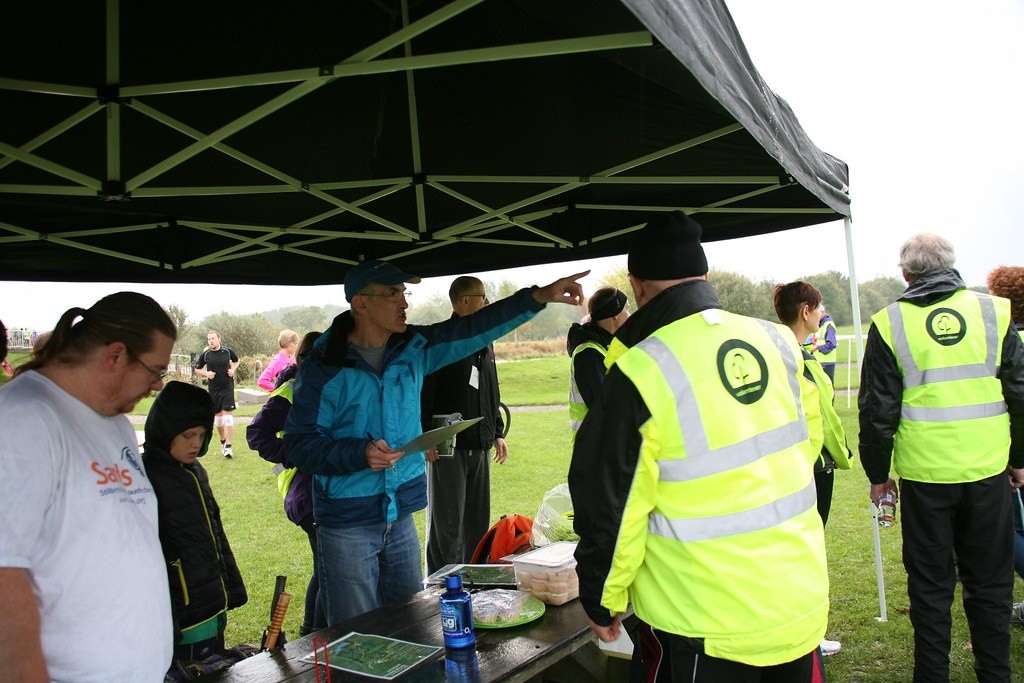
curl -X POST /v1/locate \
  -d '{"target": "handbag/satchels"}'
[529,483,578,547]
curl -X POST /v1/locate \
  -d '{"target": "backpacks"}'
[470,514,533,563]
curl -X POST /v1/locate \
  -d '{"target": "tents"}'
[0,0,888,623]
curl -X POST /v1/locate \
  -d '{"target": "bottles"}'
[439,573,475,647]
[877,487,897,527]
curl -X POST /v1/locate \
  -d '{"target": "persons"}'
[245,332,327,637]
[986,267,1024,623]
[0,292,177,683]
[418,276,508,584]
[283,260,590,627]
[136,381,248,668]
[802,300,837,406]
[576,296,596,328]
[774,280,852,656]
[259,330,301,394]
[195,330,240,458]
[858,233,1024,683]
[568,288,633,473]
[567,210,830,683]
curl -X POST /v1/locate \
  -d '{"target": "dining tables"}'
[185,581,634,683]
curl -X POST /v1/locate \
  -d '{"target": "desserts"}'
[468,588,529,624]
[518,565,579,606]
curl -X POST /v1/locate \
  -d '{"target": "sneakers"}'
[224,448,234,458]
[221,442,227,453]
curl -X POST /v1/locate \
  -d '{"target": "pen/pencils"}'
[367,431,396,472]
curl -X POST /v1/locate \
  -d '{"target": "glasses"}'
[460,292,486,303]
[355,289,412,303]
[104,340,166,383]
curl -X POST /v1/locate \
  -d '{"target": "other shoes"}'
[820,638,841,656]
[1010,601,1024,621]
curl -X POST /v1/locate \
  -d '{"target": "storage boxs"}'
[514,541,577,605]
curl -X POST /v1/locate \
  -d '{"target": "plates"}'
[469,590,545,629]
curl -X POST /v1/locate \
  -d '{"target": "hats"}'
[345,259,421,302]
[628,209,709,281]
